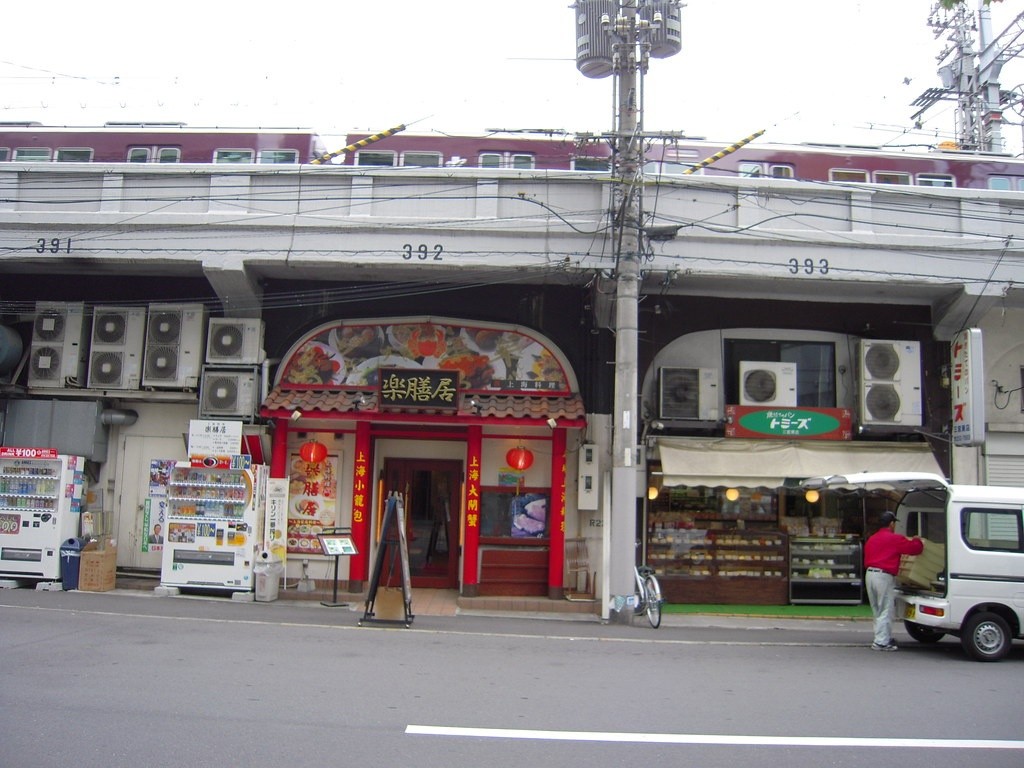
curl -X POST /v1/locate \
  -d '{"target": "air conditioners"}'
[739,361,798,407]
[28,300,90,389]
[142,302,209,388]
[198,367,262,423]
[205,317,266,364]
[87,306,145,390]
[658,366,719,422]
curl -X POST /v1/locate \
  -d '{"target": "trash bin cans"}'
[252,551,283,602]
[60,538,87,590]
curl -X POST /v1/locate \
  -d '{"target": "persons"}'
[148,524,163,544]
[864,511,924,651]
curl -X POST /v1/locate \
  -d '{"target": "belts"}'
[868,569,888,573]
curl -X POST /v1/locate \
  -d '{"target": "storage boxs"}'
[897,535,945,589]
[79,539,118,592]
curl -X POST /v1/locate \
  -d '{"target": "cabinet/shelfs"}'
[779,515,864,605]
[646,514,789,605]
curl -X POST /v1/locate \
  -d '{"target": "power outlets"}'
[859,339,923,427]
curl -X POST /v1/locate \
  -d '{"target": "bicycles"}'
[634,540,665,630]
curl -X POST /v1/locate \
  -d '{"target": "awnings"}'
[659,438,948,489]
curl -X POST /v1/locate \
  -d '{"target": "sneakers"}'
[871,637,898,651]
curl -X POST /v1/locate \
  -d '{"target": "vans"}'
[800,472,1024,661]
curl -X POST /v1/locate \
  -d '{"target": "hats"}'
[878,511,901,522]
